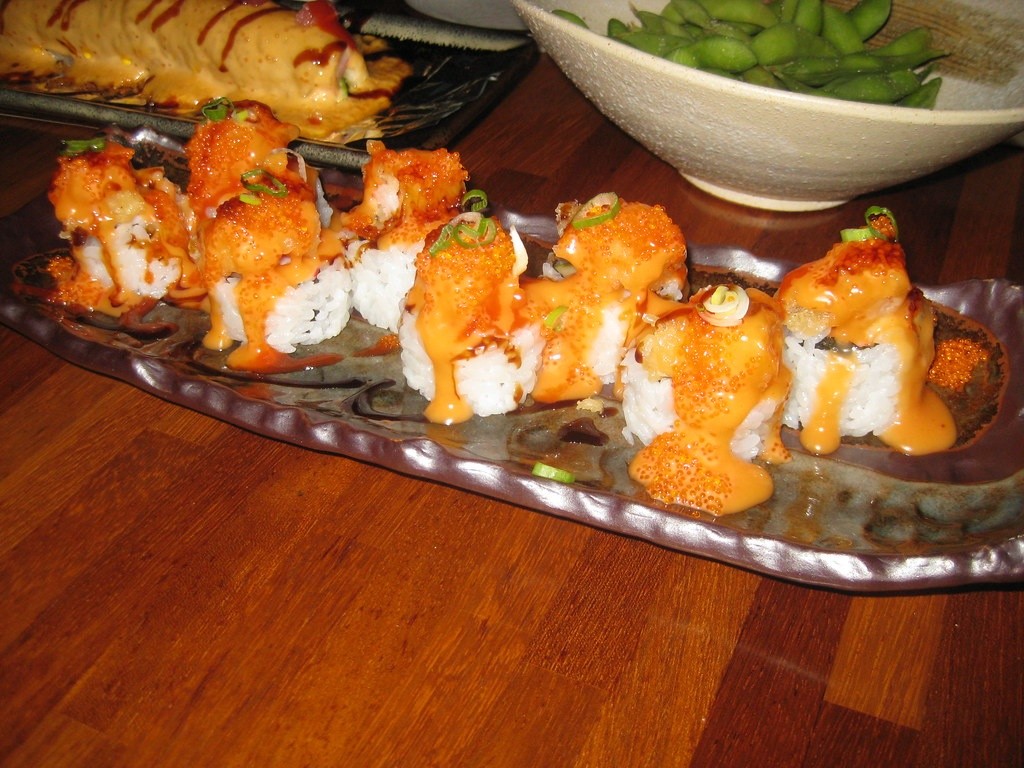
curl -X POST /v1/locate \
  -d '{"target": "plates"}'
[0,0,539,171]
[0,142,1024,593]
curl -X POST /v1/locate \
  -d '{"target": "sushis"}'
[52,100,990,515]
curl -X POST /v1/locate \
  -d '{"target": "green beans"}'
[547,0,952,111]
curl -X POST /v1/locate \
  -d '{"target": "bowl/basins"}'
[510,0,1023,213]
[408,0,531,32]
